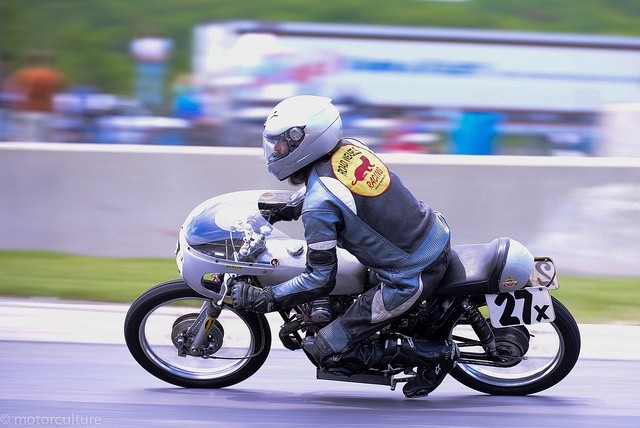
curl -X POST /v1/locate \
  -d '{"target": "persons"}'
[230,94,460,397]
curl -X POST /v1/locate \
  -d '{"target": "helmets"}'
[260,95,344,182]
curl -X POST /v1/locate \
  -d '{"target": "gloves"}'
[231,280,275,315]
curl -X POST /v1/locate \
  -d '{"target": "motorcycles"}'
[123,188,580,395]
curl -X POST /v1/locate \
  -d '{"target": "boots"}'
[386,337,460,399]
